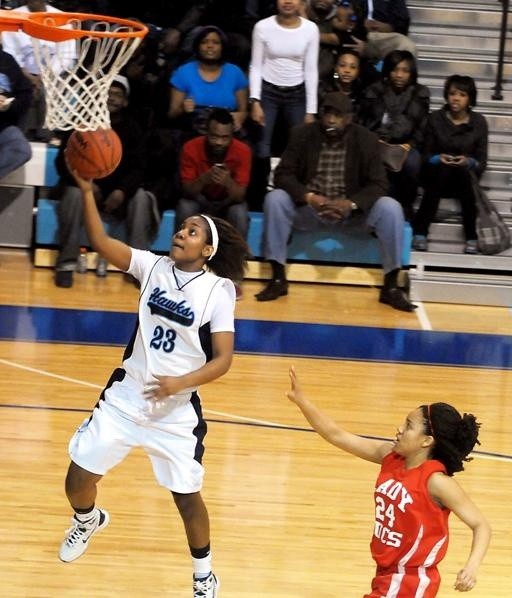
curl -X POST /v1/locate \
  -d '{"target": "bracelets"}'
[306,192,314,207]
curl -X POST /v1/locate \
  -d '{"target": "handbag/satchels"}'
[476,211,510,255]
[378,140,411,172]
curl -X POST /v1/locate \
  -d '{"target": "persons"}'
[362,49,430,231]
[58,160,254,598]
[175,110,251,297]
[412,75,490,255]
[244,2,322,213]
[53,75,151,290]
[1,2,416,182]
[286,367,491,598]
[253,94,417,310]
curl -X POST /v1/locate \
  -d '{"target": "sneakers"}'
[193,571,219,598]
[59,509,110,562]
[380,287,418,311]
[56,271,72,287]
[413,235,427,250]
[465,239,478,253]
[255,279,287,301]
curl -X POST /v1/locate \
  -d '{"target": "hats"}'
[324,91,353,113]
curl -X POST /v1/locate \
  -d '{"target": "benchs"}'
[32,53,412,293]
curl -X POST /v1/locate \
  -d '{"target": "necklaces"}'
[173,263,207,290]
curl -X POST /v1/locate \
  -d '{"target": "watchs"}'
[351,200,358,219]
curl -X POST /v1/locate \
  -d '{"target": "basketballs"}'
[68,122,122,178]
